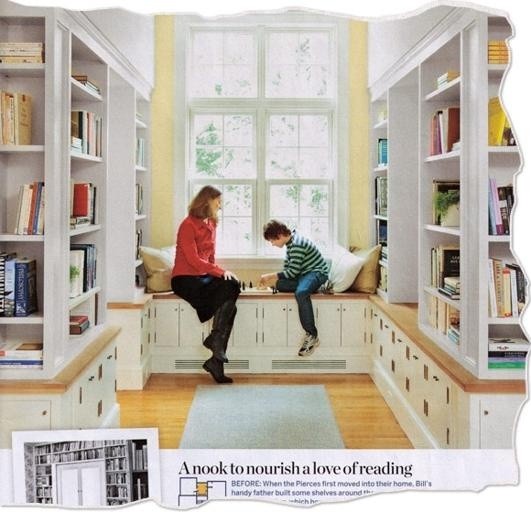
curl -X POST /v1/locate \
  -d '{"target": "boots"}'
[203,300,237,364]
[203,324,234,383]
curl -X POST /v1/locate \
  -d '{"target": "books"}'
[137,134,149,292]
[368,106,387,289]
[1,39,99,368]
[426,37,528,372]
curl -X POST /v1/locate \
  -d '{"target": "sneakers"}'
[298,332,320,357]
[317,280,334,294]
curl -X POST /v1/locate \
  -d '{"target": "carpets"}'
[177,382,347,448]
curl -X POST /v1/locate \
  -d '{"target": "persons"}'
[171,186,242,383]
[256,219,332,356]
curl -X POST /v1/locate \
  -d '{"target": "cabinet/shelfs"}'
[149,291,364,375]
